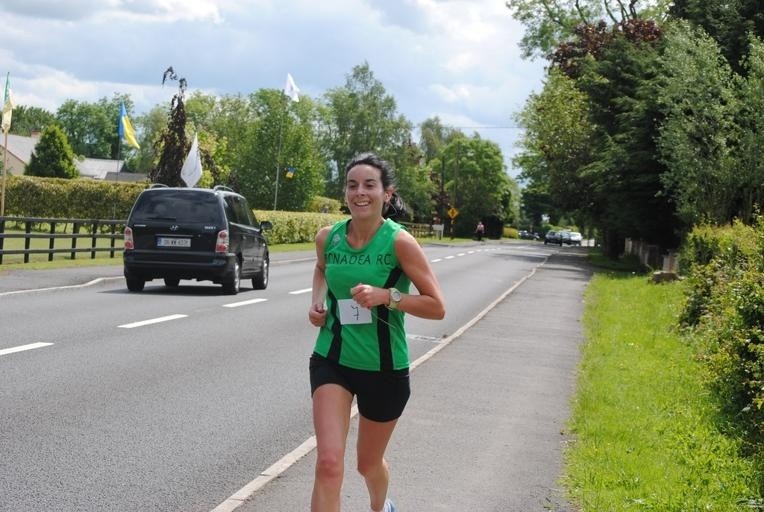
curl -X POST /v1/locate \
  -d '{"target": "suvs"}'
[123,183,273,295]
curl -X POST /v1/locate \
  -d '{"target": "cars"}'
[518,229,582,246]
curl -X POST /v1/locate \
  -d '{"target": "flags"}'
[285,74,302,104]
[119,102,143,151]
[181,135,204,188]
[285,168,297,181]
[1,74,15,134]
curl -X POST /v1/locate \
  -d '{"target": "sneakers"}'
[384,499,395,512]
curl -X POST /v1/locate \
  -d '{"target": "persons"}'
[307,152,446,512]
[475,221,486,243]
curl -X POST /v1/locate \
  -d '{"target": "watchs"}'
[386,288,403,312]
